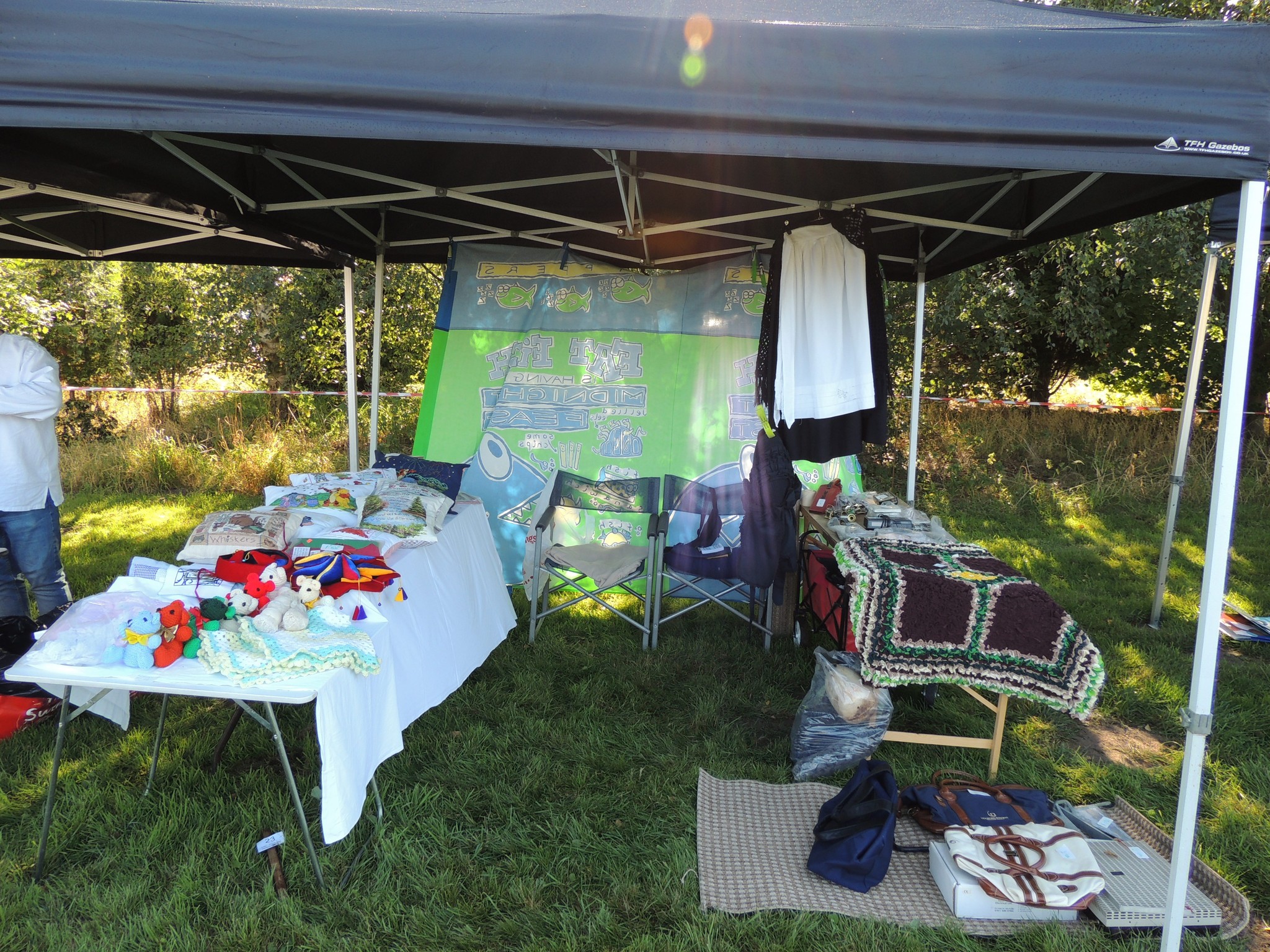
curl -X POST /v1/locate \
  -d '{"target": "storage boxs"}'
[929,836,1079,920]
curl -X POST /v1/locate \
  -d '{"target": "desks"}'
[4,458,518,901]
[789,489,1103,781]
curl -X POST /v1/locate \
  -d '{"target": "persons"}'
[0,329,74,617]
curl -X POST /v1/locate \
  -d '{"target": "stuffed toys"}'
[101,561,334,669]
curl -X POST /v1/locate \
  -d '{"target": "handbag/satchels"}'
[943,822,1105,909]
[0,600,140,741]
[893,769,1065,852]
[806,758,897,894]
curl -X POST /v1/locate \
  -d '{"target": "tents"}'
[0,0,1270,952]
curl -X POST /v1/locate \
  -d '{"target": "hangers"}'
[808,199,825,223]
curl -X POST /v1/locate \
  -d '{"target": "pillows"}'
[175,449,472,565]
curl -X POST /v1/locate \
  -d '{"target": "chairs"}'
[526,468,663,650]
[652,474,774,650]
